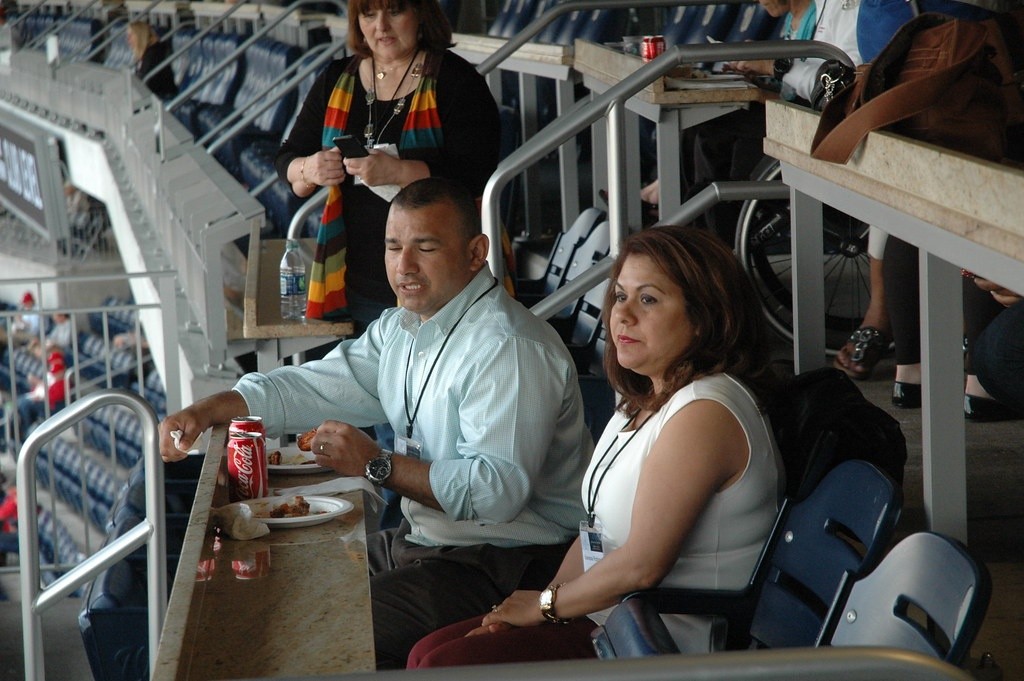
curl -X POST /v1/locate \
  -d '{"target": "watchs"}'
[539,582,575,625]
[773,57,794,81]
[365,448,393,486]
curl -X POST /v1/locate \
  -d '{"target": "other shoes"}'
[890,381,923,410]
[598,189,660,226]
[960,393,1024,424]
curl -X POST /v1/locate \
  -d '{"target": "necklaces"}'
[363,48,422,154]
[801,1,826,62]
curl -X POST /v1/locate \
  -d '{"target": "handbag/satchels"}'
[810,59,858,112]
[807,10,1024,165]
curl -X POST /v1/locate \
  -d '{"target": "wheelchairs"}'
[730,99,898,367]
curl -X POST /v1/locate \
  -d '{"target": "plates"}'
[219,496,355,529]
[680,74,745,81]
[266,447,333,475]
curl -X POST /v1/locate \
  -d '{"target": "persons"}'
[9,291,74,440]
[274,1,502,531]
[124,22,177,101]
[157,177,597,671]
[600,0,1023,423]
[390,226,789,670]
[0,478,20,568]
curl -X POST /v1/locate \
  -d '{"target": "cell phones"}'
[333,135,369,159]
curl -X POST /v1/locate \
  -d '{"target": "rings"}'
[320,442,325,455]
[491,605,498,613]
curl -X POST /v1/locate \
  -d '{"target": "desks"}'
[181,450,376,680]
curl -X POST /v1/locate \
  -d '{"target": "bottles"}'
[280,240,307,320]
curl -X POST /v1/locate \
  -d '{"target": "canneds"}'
[232,545,271,579]
[196,527,221,581]
[641,35,666,59]
[227,416,268,502]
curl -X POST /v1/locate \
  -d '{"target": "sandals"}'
[832,324,894,381]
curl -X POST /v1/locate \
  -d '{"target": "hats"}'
[48,360,66,373]
[46,352,65,363]
[19,292,35,306]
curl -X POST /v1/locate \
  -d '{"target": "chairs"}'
[734,422,993,668]
[4,0,783,597]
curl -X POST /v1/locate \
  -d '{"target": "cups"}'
[623,37,641,58]
